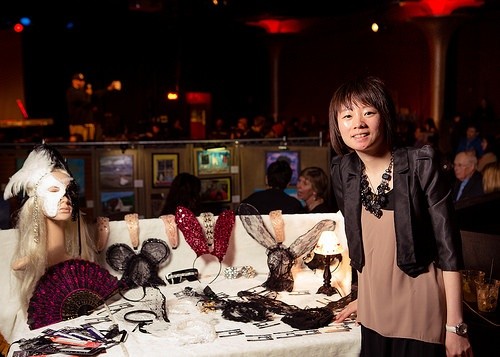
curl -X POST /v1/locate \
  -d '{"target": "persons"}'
[396,103,500,234]
[67,115,331,150]
[12,169,77,271]
[160,160,339,217]
[329,77,472,357]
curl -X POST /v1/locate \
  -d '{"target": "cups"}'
[461,270,485,302]
[474,278,500,313]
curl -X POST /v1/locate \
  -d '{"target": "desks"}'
[6,269,363,357]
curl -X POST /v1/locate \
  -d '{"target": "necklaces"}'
[360,157,394,219]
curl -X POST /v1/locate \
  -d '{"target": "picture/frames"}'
[151,152,179,189]
[199,176,232,203]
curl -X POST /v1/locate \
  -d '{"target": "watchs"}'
[446,323,467,336]
